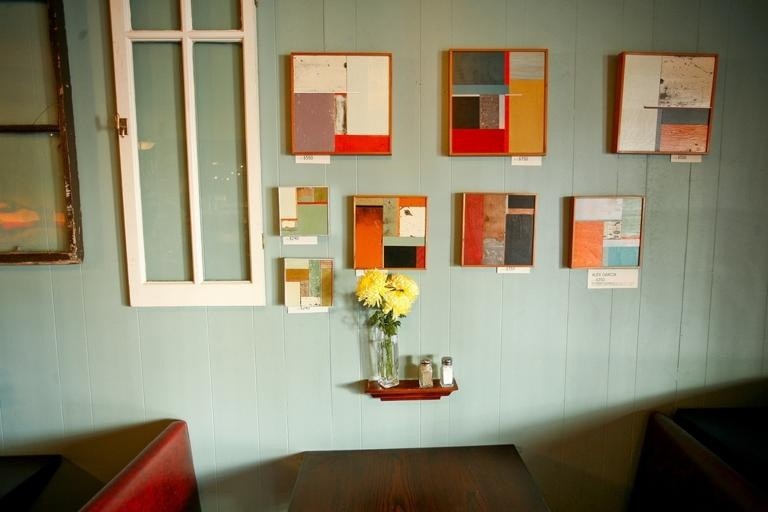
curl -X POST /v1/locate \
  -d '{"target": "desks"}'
[286,442,556,512]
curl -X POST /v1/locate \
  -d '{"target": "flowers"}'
[352,267,420,381]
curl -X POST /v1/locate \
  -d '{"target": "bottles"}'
[439,356,454,388]
[418,359,435,389]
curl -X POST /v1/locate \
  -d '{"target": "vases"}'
[367,326,402,390]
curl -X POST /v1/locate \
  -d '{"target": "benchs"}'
[624,410,766,511]
[77,418,203,511]
